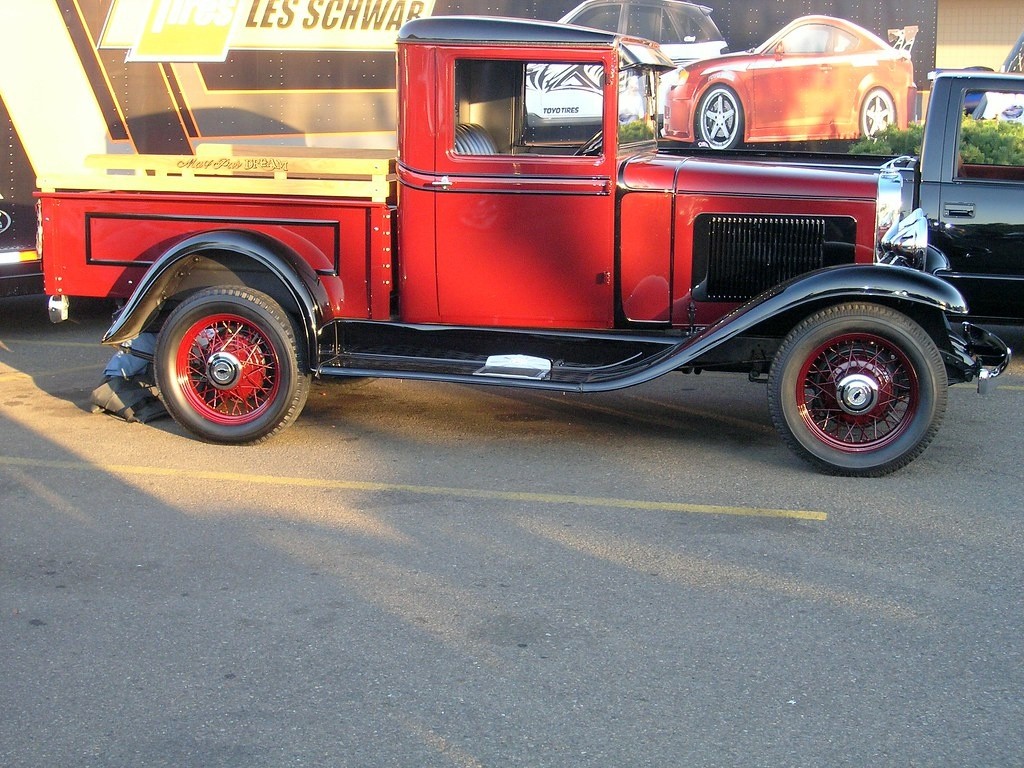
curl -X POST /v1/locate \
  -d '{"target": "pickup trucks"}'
[654,65,1024,355]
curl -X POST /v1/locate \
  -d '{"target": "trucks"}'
[32,12,1012,478]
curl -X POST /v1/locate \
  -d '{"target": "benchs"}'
[453,122,499,155]
